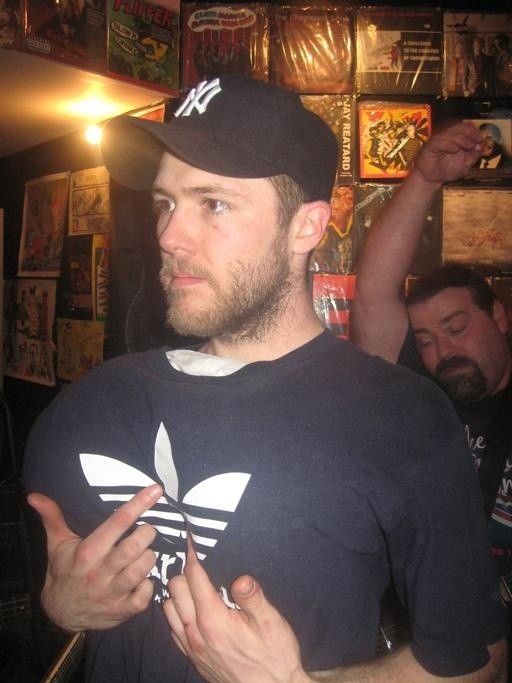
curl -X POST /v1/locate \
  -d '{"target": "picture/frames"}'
[355,98,435,184]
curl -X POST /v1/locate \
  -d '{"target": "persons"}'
[467,124,509,170]
[193,38,253,80]
[346,118,511,681]
[21,81,512,683]
[365,119,425,173]
[462,31,512,98]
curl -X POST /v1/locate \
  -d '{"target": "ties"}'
[483,161,489,168]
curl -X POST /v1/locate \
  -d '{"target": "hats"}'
[100,77,338,209]
